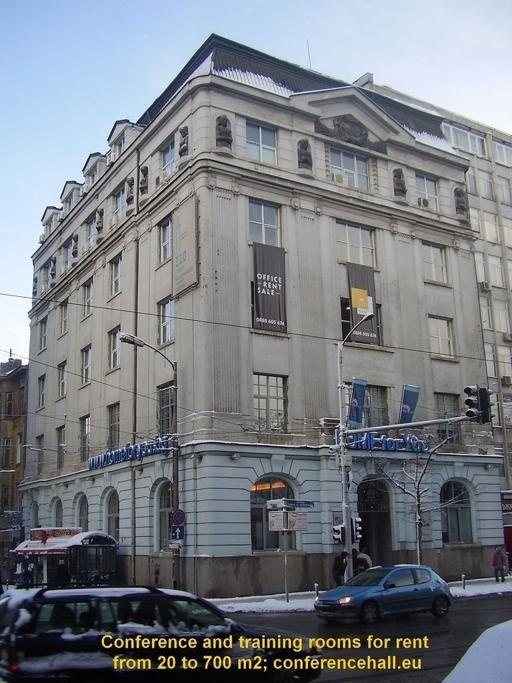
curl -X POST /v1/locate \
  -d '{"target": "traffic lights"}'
[352,516,363,546]
[479,385,496,423]
[463,382,484,423]
[332,524,345,544]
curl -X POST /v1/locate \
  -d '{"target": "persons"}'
[492,546,507,582]
[357,548,372,568]
[332,552,348,586]
[22,555,34,585]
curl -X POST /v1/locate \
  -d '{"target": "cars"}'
[310,563,454,625]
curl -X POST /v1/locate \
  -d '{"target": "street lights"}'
[117,331,185,591]
[335,309,378,581]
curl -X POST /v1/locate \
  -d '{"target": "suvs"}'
[0,580,326,682]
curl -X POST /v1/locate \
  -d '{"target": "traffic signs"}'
[283,499,314,509]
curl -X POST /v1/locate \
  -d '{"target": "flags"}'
[349,378,368,426]
[400,383,421,423]
